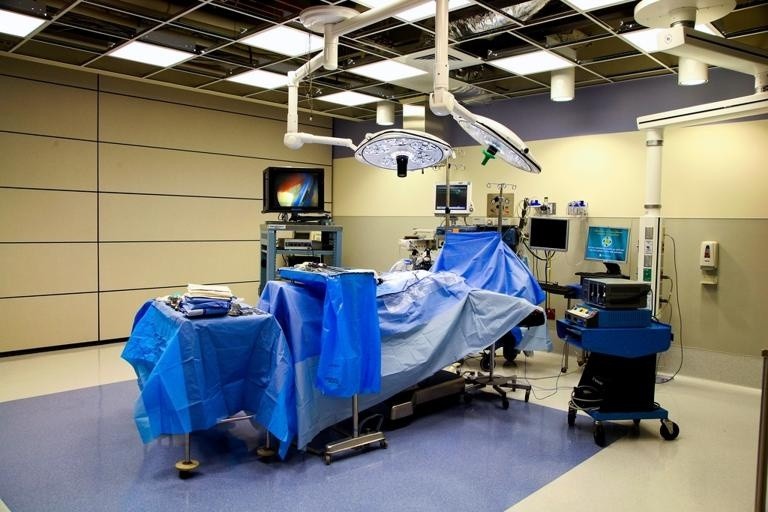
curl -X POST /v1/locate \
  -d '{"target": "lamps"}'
[280,55,454,179]
[546,56,580,101]
[429,0,542,176]
[375,99,397,128]
[676,57,710,87]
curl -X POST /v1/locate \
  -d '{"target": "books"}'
[187,284,232,300]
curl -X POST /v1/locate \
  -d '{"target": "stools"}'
[461,290,544,411]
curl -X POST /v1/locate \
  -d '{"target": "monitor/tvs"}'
[584,225,631,275]
[261,167,324,222]
[528,216,569,252]
[433,181,471,214]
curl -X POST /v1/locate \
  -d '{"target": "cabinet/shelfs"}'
[260,223,343,300]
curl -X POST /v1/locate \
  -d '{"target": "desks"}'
[149,299,282,480]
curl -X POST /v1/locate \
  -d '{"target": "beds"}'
[260,272,476,465]
